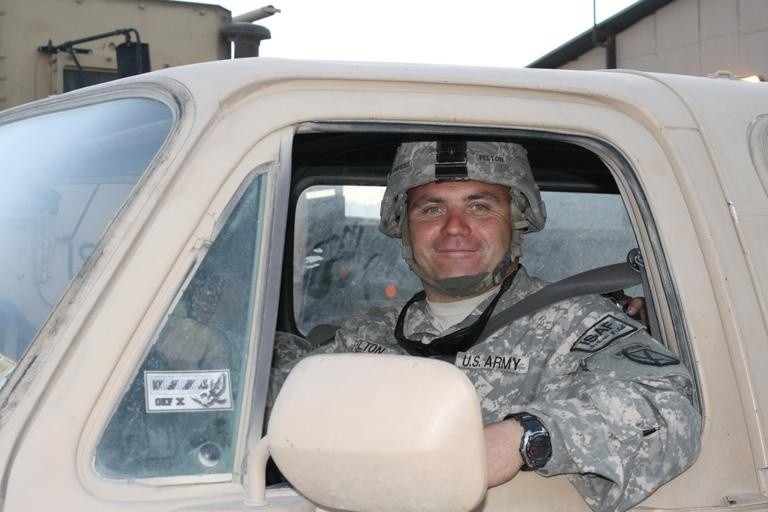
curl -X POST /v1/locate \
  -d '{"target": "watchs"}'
[504,411,551,472]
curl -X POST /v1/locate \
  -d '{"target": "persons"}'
[156,133,704,511]
[100,233,318,468]
[602,289,647,322]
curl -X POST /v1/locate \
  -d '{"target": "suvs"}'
[0,59,768,512]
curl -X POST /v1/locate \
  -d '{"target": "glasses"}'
[395,287,505,357]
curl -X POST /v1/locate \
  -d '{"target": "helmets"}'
[380,141,546,239]
